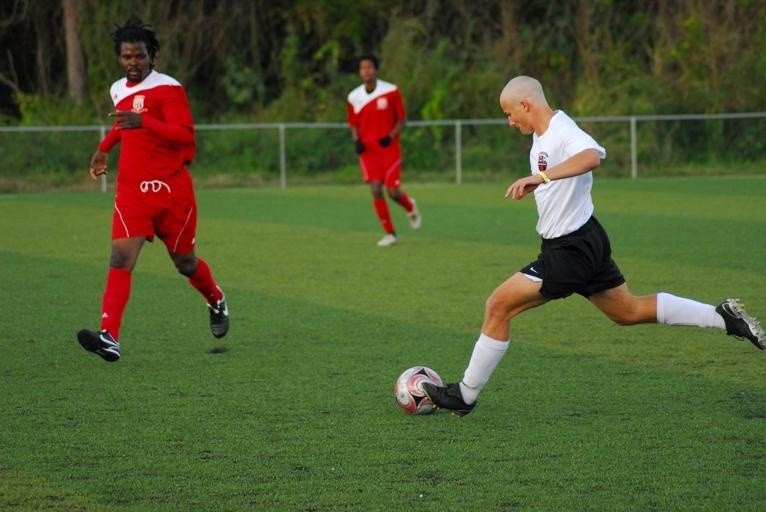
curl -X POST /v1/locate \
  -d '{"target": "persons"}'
[346,53,421,248]
[75,14,229,362]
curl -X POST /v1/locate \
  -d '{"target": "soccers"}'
[394,365,442,414]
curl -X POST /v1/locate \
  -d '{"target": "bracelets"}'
[536,171,551,184]
[419,74,766,419]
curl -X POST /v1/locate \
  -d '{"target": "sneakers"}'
[406,197,422,231]
[377,234,399,247]
[207,285,231,338]
[714,297,766,352]
[422,381,478,420]
[76,328,121,362]
